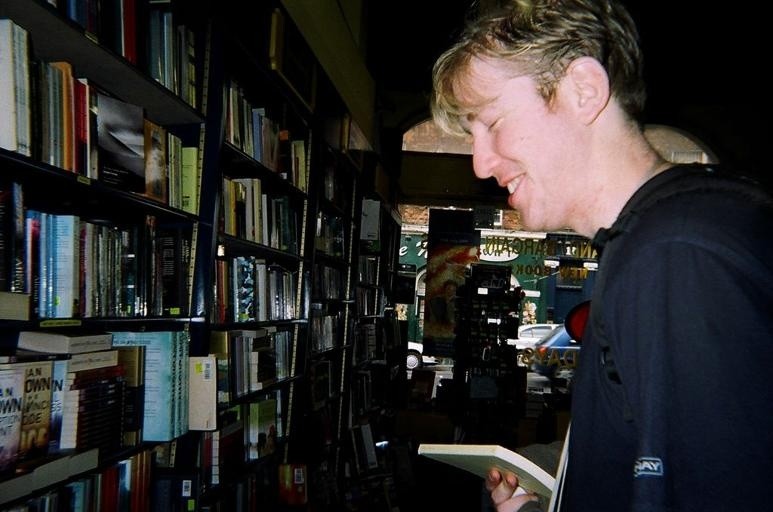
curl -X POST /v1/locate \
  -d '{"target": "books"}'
[313,303,351,350]
[344,372,380,494]
[13,439,177,511]
[218,250,302,321]
[220,68,309,196]
[314,265,346,301]
[316,155,351,209]
[208,327,300,398]
[312,210,347,263]
[419,440,558,499]
[220,163,306,260]
[0,320,225,459]
[1,180,201,321]
[353,255,390,286]
[353,322,374,370]
[180,387,296,500]
[307,356,338,446]
[27,0,205,111]
[356,190,388,252]
[1,18,200,216]
[184,448,328,511]
[352,288,390,319]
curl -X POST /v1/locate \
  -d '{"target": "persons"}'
[430,1,770,512]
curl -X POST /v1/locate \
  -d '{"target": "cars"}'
[505,324,561,363]
[534,324,582,376]
[406,339,440,369]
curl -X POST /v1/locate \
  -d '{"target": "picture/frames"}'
[468,262,512,292]
